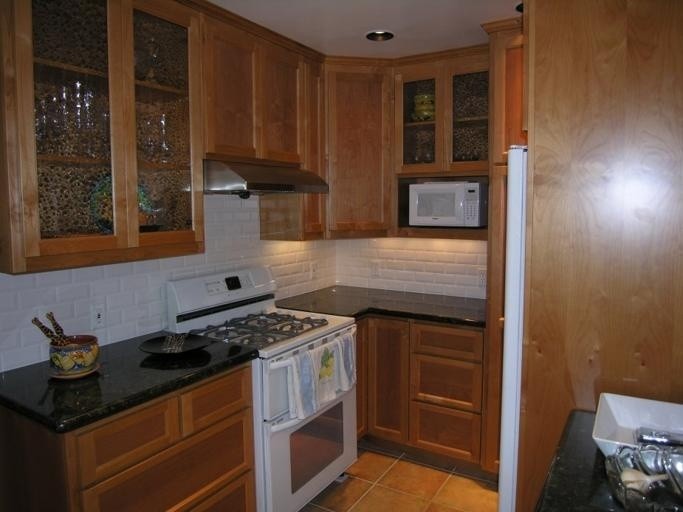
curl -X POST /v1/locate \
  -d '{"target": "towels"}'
[287,333,359,418]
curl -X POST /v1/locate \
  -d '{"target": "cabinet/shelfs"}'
[200,1,305,166]
[0,1,206,276]
[520,1,681,149]
[479,15,525,472]
[391,52,490,240]
[367,314,409,456]
[357,315,367,440]
[405,318,485,475]
[301,43,321,237]
[0,330,259,512]
[324,57,394,241]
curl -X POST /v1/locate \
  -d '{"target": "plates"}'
[137,332,227,354]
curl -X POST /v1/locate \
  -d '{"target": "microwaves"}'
[408,181,488,227]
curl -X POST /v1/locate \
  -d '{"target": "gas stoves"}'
[168,294,356,358]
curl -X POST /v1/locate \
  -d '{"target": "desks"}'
[531,409,682,512]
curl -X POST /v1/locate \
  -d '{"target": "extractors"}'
[203,159,329,198]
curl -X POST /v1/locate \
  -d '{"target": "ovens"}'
[252,324,357,512]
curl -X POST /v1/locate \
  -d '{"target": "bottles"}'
[35,81,111,156]
